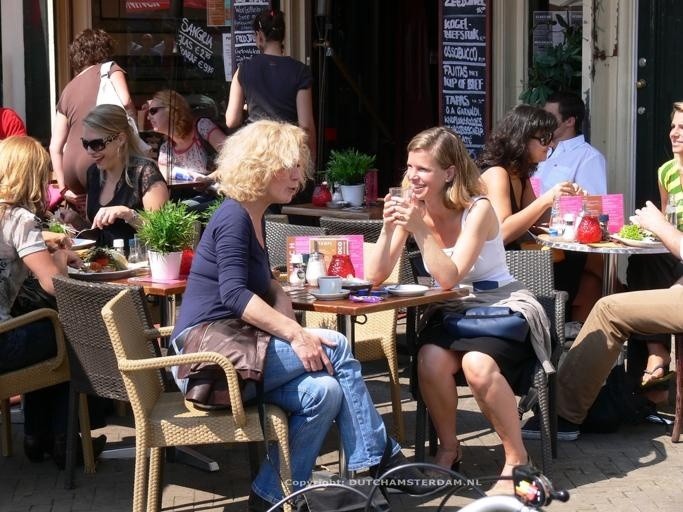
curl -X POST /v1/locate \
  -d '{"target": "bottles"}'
[312,184,332,207]
[287,251,355,287]
[547,193,609,245]
[111,238,143,266]
[663,193,678,228]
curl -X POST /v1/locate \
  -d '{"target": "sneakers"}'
[519,416,580,441]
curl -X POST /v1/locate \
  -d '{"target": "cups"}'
[316,277,342,293]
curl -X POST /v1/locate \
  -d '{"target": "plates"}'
[308,284,427,300]
[69,267,137,279]
[610,232,662,251]
[69,238,95,249]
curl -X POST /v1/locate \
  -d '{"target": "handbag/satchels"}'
[441,307,528,343]
[293,477,391,512]
[96,61,139,138]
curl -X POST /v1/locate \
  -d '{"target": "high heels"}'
[369,450,447,505]
[423,441,462,480]
[484,456,532,496]
[248,489,284,512]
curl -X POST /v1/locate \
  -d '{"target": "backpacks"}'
[517,321,623,433]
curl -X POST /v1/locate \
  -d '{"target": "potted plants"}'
[316,148,376,208]
[128,199,204,281]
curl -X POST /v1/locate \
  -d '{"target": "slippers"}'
[638,365,674,389]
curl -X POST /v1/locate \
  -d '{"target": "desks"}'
[278,199,384,220]
[160,178,207,200]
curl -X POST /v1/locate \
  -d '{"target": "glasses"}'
[82,133,119,151]
[528,132,552,145]
[150,107,166,116]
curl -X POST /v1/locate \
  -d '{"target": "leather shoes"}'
[23,432,106,464]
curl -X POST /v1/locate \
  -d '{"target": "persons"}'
[363,124,553,506]
[165,116,453,512]
[2,0,683,466]
